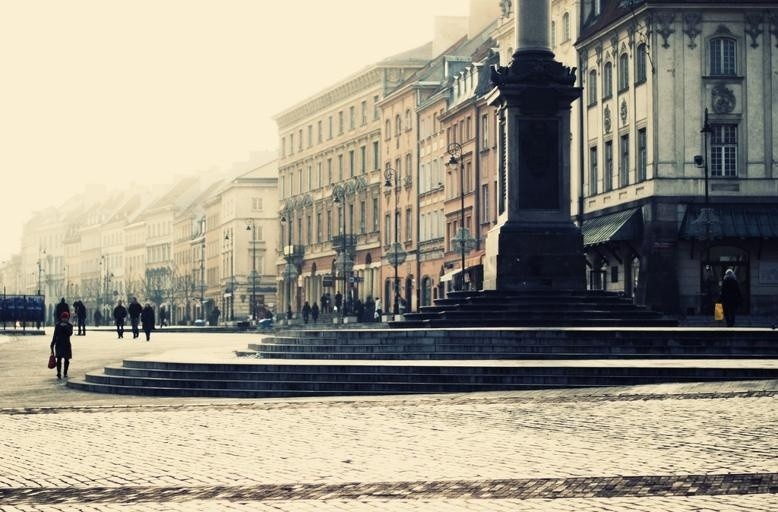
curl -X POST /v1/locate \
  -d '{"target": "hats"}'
[61,312,69,318]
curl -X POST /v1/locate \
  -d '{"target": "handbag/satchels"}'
[48,352,56,369]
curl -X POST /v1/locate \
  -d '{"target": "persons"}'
[53,297,70,324]
[93,311,101,327]
[128,297,143,338]
[158,305,168,328]
[140,302,156,341]
[73,300,86,336]
[49,311,72,378]
[375,297,383,323]
[111,299,127,337]
[302,291,343,324]
[720,268,741,327]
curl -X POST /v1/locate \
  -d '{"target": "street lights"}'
[98,255,113,325]
[224,222,234,322]
[332,185,348,324]
[447,142,467,290]
[245,217,257,321]
[382,168,400,320]
[199,238,205,320]
[278,209,293,321]
[692,106,716,272]
